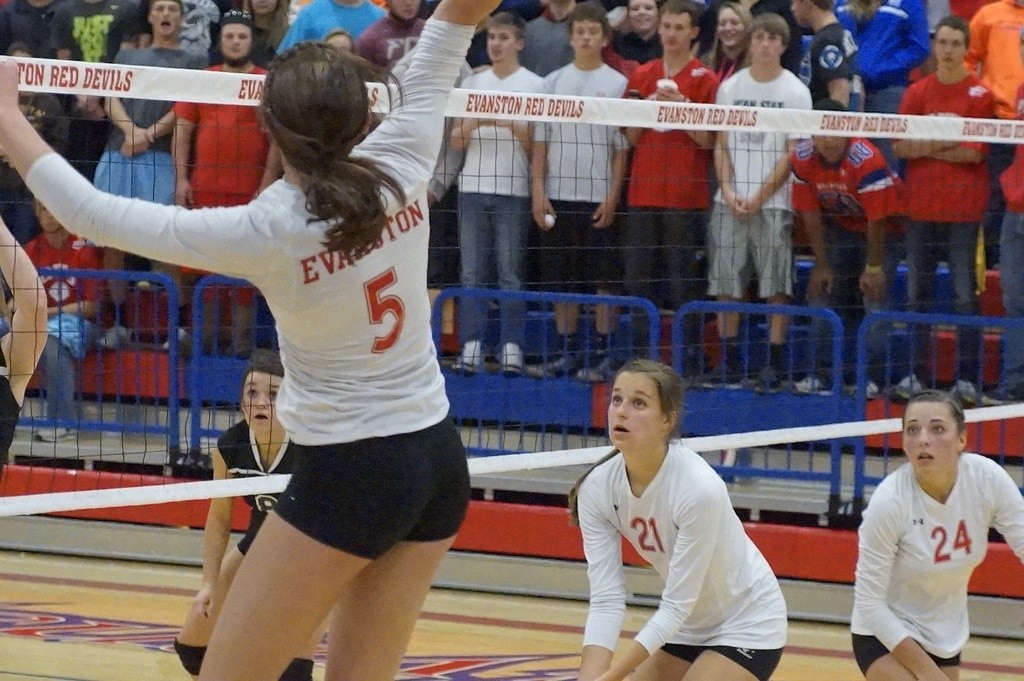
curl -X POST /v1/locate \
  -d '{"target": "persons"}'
[566,359,787,681]
[174,346,330,681]
[0,0,1024,442]
[850,389,1024,681]
[0,0,501,681]
[0,216,48,479]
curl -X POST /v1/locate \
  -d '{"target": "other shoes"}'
[98,325,134,349]
[792,377,835,397]
[501,343,524,374]
[577,357,615,382]
[162,327,190,351]
[982,389,1023,404]
[527,356,568,378]
[702,373,746,390]
[849,380,880,399]
[755,374,789,393]
[450,341,482,372]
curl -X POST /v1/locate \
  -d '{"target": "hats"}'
[219,9,253,28]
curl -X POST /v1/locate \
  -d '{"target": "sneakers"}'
[890,374,923,398]
[36,426,79,442]
[951,380,977,405]
